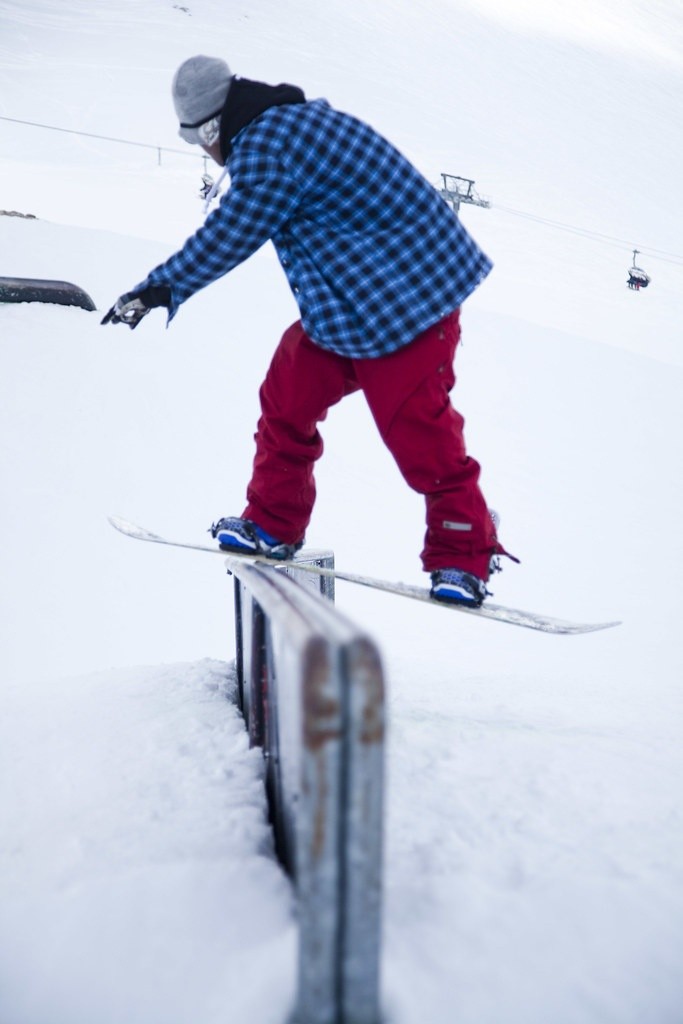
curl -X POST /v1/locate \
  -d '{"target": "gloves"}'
[102,292,154,332]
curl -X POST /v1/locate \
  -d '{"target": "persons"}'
[101,54,522,608]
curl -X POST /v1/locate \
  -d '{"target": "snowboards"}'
[102,509,631,637]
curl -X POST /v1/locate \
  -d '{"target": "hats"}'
[173,55,233,146]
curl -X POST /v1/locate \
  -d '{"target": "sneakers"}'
[431,568,487,607]
[211,517,303,562]
